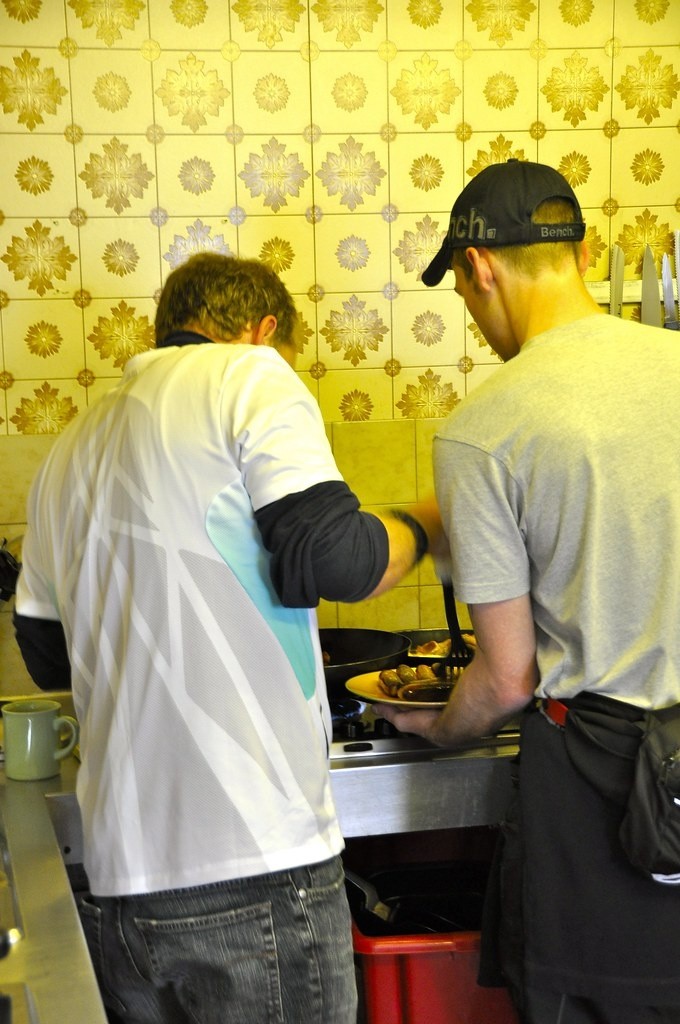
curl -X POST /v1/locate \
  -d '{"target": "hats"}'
[421,157,586,287]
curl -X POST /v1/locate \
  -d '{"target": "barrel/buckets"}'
[353,853,525,1024]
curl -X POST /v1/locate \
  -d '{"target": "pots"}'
[316,628,412,696]
[392,629,480,665]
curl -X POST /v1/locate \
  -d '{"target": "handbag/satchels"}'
[620,716,680,887]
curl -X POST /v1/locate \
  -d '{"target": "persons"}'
[12,253,448,1024]
[373,158,679,1024]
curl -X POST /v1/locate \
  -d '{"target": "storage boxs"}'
[348,923,528,1024]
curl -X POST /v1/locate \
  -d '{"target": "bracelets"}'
[390,508,429,569]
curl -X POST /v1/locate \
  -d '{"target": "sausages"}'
[378,662,458,702]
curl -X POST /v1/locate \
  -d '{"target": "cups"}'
[1,699,80,780]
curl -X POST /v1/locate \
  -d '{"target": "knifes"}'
[662,252,679,330]
[674,229,680,329]
[640,244,660,327]
[610,244,625,318]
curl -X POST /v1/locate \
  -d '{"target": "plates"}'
[345,667,467,708]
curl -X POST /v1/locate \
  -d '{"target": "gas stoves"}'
[329,699,521,839]
